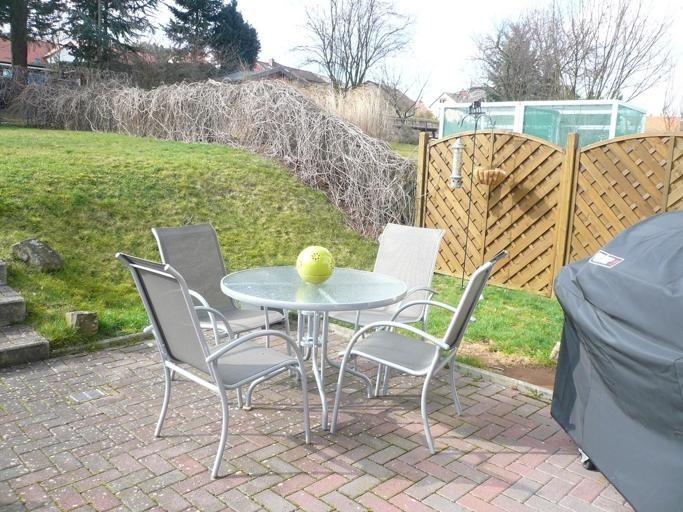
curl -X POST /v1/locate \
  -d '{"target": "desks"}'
[216,261,417,432]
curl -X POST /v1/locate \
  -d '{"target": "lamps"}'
[447,137,471,193]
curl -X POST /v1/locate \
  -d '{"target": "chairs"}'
[328,248,513,457]
[148,221,298,408]
[112,250,315,483]
[306,222,448,400]
